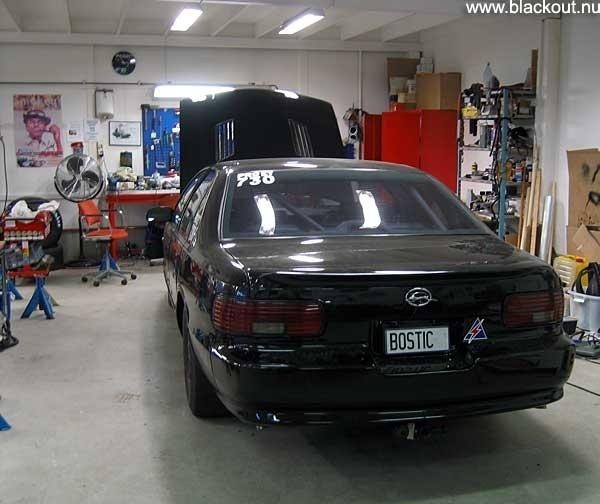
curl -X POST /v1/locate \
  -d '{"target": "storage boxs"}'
[416,73,461,110]
[568,286,600,332]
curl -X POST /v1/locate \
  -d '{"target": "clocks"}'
[112,51,137,74]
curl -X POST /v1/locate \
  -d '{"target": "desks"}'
[105,190,182,261]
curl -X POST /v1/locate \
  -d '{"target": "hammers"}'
[141,104,159,132]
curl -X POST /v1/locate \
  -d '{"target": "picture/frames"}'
[109,120,141,146]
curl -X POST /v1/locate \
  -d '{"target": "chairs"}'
[77,199,136,287]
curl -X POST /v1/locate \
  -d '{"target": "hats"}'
[23,110,50,125]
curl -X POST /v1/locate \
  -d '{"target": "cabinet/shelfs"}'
[454,88,533,239]
[365,109,459,196]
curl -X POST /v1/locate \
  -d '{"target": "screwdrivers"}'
[145,143,149,170]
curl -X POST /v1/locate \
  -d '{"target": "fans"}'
[53,154,105,267]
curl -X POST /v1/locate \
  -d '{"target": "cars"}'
[154,84,576,443]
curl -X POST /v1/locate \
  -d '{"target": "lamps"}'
[279,7,326,34]
[168,3,203,32]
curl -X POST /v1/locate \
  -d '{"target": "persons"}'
[16,104,63,166]
